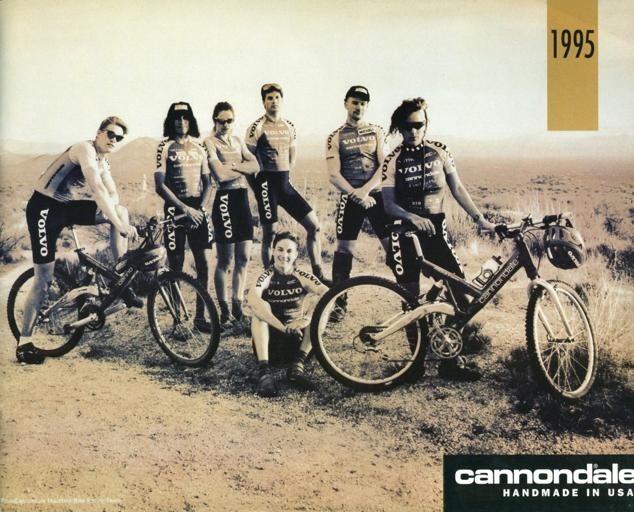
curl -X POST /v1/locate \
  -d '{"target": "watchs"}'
[200,205,206,215]
[473,213,483,223]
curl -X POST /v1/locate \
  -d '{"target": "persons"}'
[156,103,222,340]
[245,84,335,291]
[325,86,410,322]
[381,97,506,381]
[247,232,335,397]
[203,102,259,329]
[16,116,138,365]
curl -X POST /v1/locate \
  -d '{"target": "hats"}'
[170,102,193,114]
[345,85,370,102]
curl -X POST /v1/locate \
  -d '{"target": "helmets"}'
[542,226,587,270]
[125,246,167,272]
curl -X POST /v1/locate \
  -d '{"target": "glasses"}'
[104,129,124,142]
[402,121,423,130]
[263,84,281,90]
[215,118,233,124]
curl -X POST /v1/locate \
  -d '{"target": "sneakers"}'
[122,287,143,308]
[172,328,188,341]
[256,366,276,395]
[287,351,307,381]
[331,306,345,321]
[16,343,44,364]
[194,318,212,332]
[438,361,481,381]
[237,315,252,328]
[219,317,234,337]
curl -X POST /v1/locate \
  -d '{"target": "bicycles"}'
[310,212,598,400]
[7,217,219,367]
